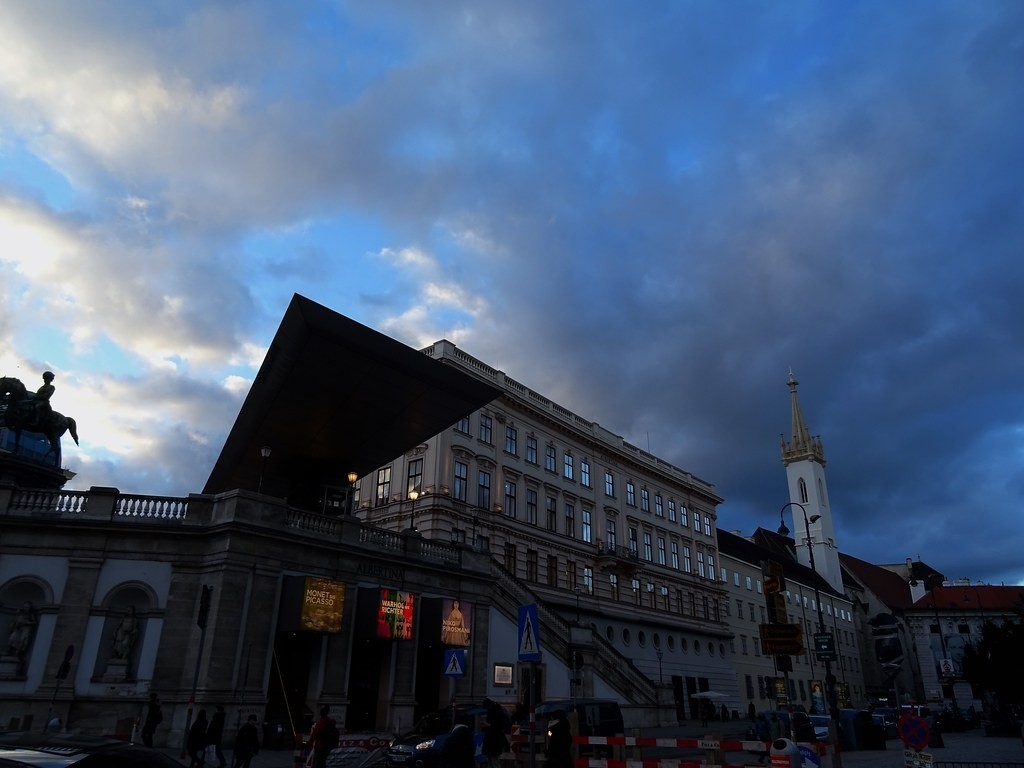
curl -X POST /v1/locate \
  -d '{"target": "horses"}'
[0,378,79,468]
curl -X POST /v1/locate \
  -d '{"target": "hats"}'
[248,715,257,721]
[320,704,330,716]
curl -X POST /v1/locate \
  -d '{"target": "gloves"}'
[304,748,310,756]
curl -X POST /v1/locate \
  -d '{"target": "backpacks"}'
[318,720,339,748]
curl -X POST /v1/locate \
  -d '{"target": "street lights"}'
[574,586,582,625]
[258,445,273,493]
[346,471,358,518]
[962,584,985,631]
[469,507,480,545]
[656,648,666,683]
[778,502,844,768]
[409,490,419,530]
[907,573,964,733]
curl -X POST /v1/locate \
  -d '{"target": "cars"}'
[0,730,188,768]
[808,714,832,742]
[901,705,934,728]
[385,699,513,768]
[745,711,814,746]
[871,715,898,739]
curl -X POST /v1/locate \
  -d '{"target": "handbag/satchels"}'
[206,744,215,762]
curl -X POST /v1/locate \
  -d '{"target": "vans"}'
[516,696,624,755]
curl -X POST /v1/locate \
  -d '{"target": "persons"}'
[138,692,164,748]
[186,709,209,768]
[5,601,38,657]
[700,701,730,728]
[479,699,506,768]
[748,702,756,720]
[515,699,529,725]
[30,372,54,426]
[305,705,340,768]
[201,704,227,768]
[111,604,140,663]
[232,714,260,768]
[541,711,574,768]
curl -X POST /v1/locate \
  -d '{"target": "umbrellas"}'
[691,690,729,699]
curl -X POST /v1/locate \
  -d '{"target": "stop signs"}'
[944,664,952,670]
